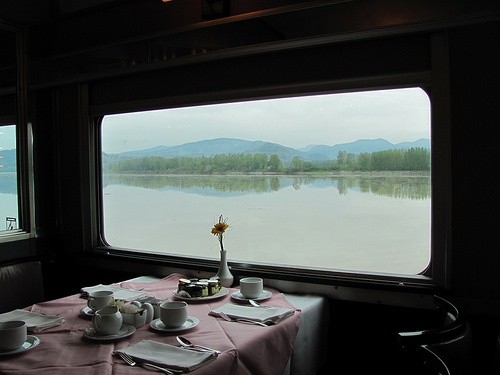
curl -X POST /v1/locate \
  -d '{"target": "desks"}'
[0,276,323,375]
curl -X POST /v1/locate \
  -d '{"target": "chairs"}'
[0,256,64,312]
[397,295,467,375]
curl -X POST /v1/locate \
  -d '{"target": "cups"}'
[159,301,189,328]
[92,305,123,335]
[240,277,263,298]
[0,321,27,350]
[87,291,114,311]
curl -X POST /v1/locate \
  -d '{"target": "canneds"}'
[178,276,220,298]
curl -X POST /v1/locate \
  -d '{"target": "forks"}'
[218,311,268,327]
[118,351,174,375]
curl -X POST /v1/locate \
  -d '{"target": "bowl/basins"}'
[121,309,147,328]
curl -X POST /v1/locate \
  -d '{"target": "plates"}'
[149,316,200,331]
[231,290,272,302]
[173,287,229,300]
[0,335,40,355]
[80,306,95,318]
[83,324,136,340]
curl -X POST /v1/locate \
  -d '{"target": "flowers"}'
[210,214,229,250]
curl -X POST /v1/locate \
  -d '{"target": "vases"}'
[216,250,234,287]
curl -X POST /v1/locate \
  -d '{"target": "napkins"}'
[112,339,214,373]
[79,284,148,302]
[208,303,295,323]
[0,309,64,334]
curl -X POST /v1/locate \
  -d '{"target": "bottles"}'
[178,277,221,298]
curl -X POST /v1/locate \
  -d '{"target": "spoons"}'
[249,299,261,306]
[175,335,221,354]
